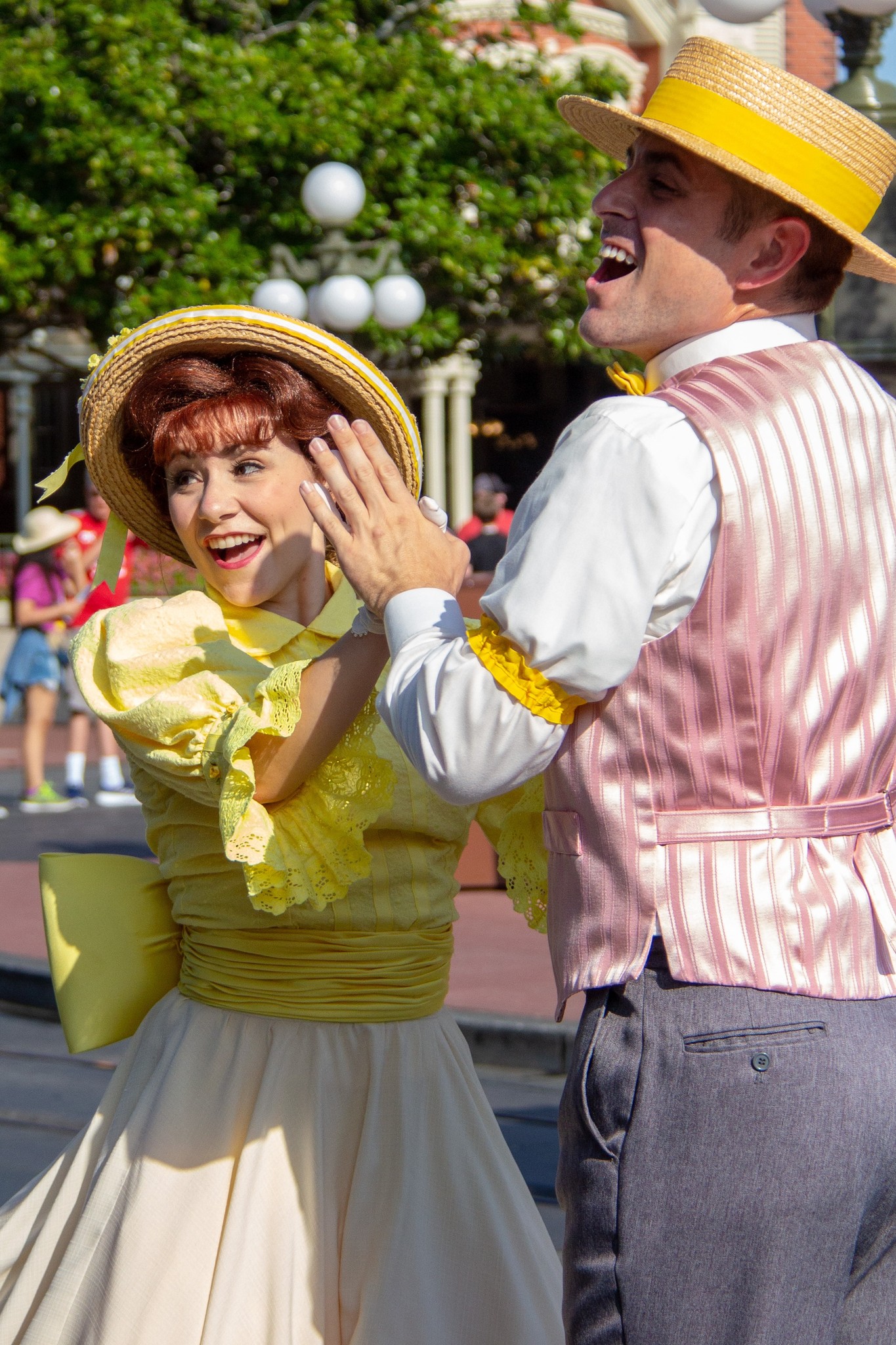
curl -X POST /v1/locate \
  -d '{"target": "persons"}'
[0,469,153,815]
[295,36,896,1345]
[455,472,516,578]
[0,302,564,1345]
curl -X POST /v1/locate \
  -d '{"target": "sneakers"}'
[94,783,142,807]
[65,781,90,806]
[19,780,73,813]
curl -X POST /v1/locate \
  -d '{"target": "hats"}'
[12,506,82,556]
[76,304,424,569]
[556,37,896,284]
